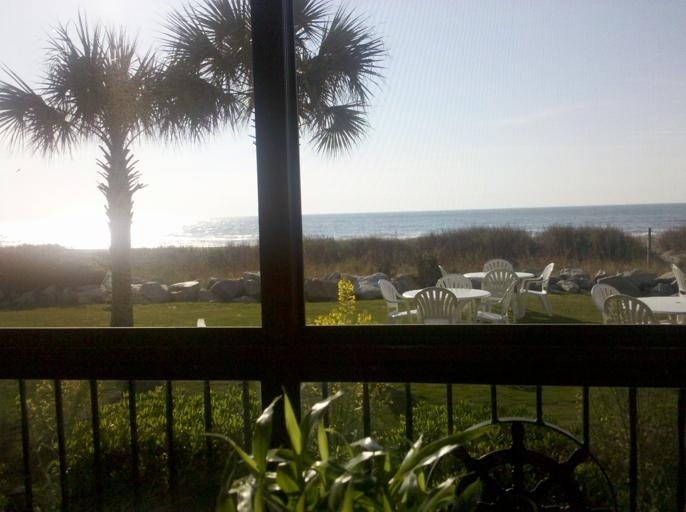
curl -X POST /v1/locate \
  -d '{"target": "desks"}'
[620,295,686,324]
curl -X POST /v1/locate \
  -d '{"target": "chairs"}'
[591,283,622,312]
[672,263,686,296]
[379,259,554,326]
[604,295,673,325]
